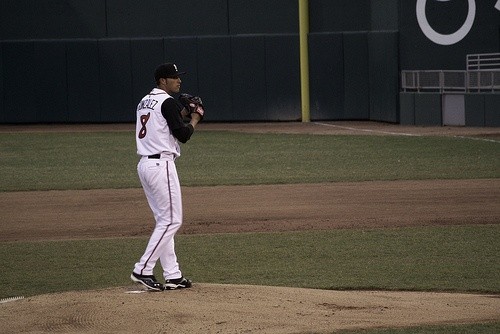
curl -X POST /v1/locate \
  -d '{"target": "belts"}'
[148,154,161,159]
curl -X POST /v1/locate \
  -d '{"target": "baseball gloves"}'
[179,94,206,121]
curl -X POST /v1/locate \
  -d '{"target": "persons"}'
[131,62,206,292]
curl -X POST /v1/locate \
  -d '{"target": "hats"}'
[153,62,186,79]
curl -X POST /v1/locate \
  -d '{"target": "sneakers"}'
[131,272,164,291]
[166,277,191,290]
